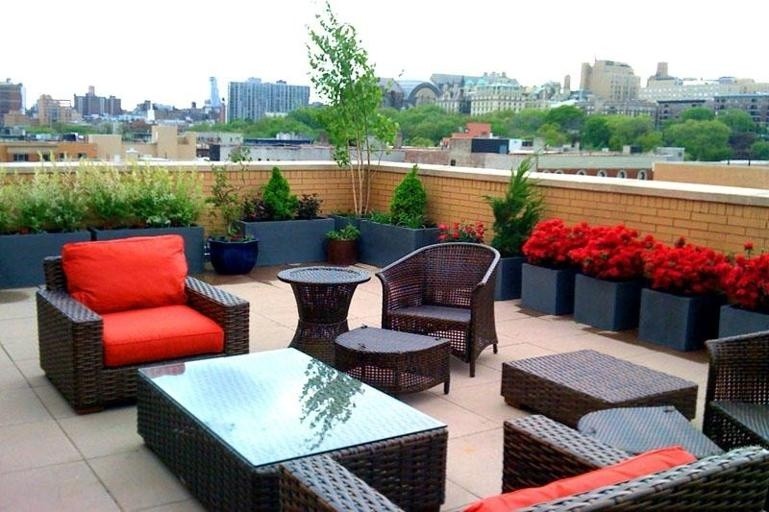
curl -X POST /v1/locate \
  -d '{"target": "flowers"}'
[638,236,734,302]
[570,222,659,279]
[719,240,769,313]
[521,217,591,271]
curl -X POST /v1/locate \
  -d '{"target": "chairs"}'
[702,331,768,452]
[35,232,250,414]
[375,242,501,377]
[277,413,768,512]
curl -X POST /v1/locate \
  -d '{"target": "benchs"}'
[500,348,698,430]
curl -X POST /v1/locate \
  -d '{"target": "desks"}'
[276,265,371,367]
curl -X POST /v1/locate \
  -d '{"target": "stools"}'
[577,405,727,459]
[333,324,450,395]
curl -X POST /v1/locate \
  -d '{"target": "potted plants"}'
[78,158,206,278]
[480,147,549,304]
[204,164,260,276]
[282,0,407,237]
[0,149,93,291]
[357,163,441,270]
[326,223,362,266]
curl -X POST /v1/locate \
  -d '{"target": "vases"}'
[574,273,644,334]
[718,305,769,339]
[640,286,714,354]
[521,261,578,317]
